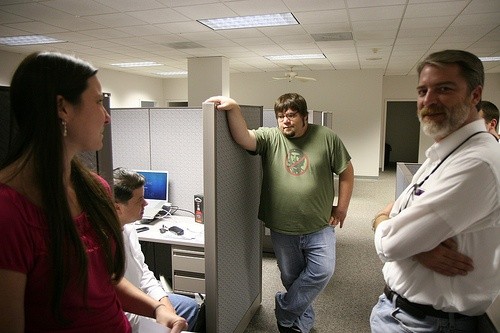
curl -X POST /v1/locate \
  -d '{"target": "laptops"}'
[133,170,168,219]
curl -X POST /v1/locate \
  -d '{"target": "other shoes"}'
[275,292,301,333]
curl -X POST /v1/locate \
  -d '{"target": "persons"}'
[0,51,189,333]
[370,49,500,333]
[477,100,500,143]
[203,93,354,333]
[112,166,200,331]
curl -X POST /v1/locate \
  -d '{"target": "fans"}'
[271,67,316,83]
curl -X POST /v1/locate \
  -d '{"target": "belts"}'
[382,285,480,320]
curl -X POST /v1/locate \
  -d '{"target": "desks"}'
[131,213,205,298]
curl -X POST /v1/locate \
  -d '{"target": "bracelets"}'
[153,303,165,316]
[372,212,390,231]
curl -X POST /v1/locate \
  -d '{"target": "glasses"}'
[278,111,298,121]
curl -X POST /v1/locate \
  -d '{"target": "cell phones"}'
[136,227,149,233]
